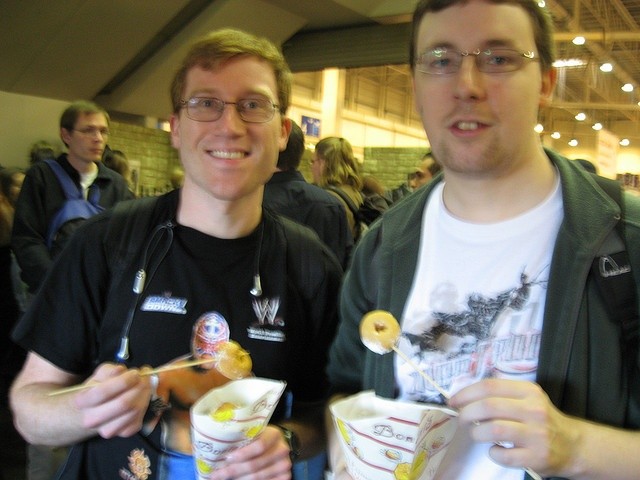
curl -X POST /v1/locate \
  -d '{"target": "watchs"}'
[278,424,301,465]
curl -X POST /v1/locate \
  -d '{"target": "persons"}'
[310,134,363,239]
[8,29,342,480]
[1,167,28,246]
[12,101,138,293]
[409,153,443,194]
[263,119,353,274]
[326,1,638,480]
[104,149,135,193]
[28,139,56,164]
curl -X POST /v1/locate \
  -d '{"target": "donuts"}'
[212,401,238,413]
[213,339,253,381]
[358,309,402,356]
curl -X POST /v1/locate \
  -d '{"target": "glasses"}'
[308,158,324,166]
[412,51,542,75]
[72,127,111,136]
[176,98,281,123]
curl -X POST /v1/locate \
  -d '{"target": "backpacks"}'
[322,185,389,243]
[43,160,106,259]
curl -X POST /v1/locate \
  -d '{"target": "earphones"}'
[249,275,262,297]
[117,338,131,360]
